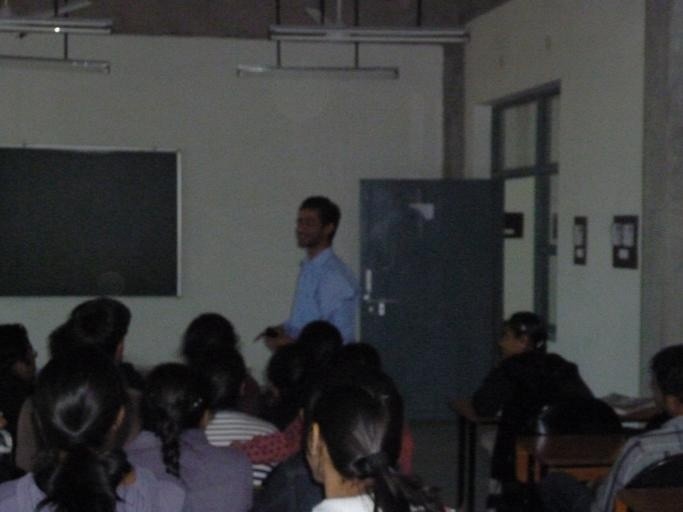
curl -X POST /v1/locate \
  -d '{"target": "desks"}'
[517,432,640,511]
[617,486,683,512]
[447,399,519,512]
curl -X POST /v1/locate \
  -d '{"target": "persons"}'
[538,342,683,512]
[260,196,360,347]
[453,306,593,482]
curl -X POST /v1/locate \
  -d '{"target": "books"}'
[600,391,657,415]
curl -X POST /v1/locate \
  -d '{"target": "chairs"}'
[527,396,624,434]
[625,451,682,488]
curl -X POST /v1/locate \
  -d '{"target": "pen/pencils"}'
[250,323,286,343]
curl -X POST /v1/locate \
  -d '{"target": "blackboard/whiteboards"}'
[0,145,184,298]
[358,177,505,427]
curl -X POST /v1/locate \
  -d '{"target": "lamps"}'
[237,0,470,82]
[0,1,112,74]
